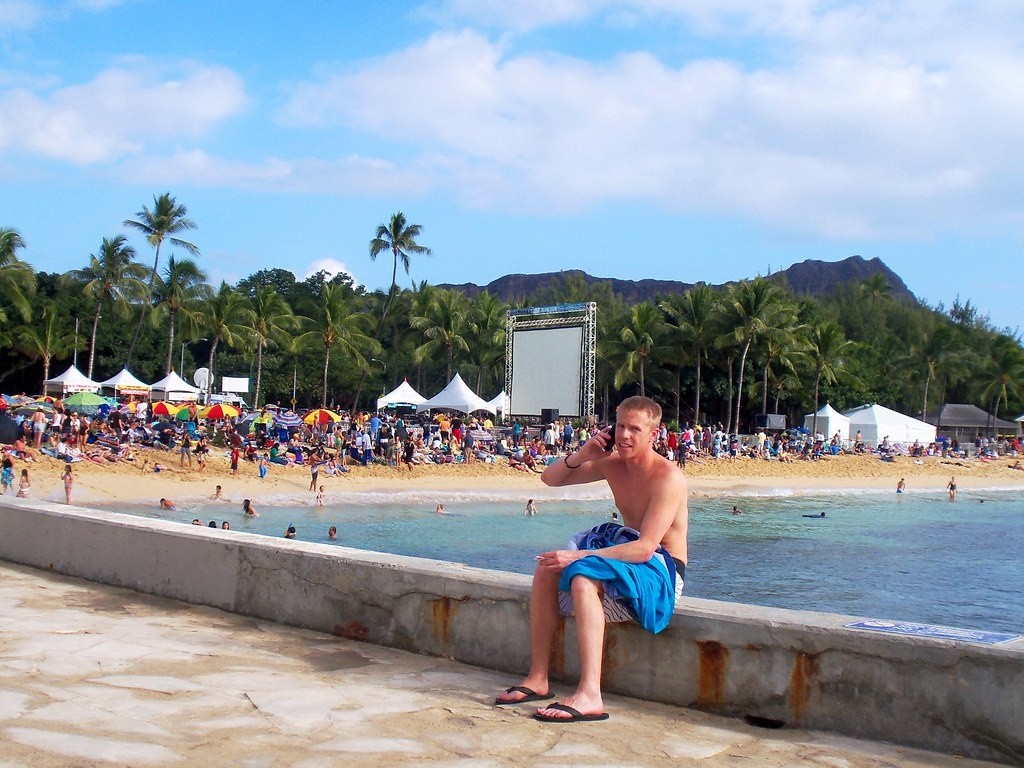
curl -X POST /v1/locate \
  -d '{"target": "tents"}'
[803,403,849,441]
[377,373,512,415]
[838,404,937,444]
[43,365,208,395]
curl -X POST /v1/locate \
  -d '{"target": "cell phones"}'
[600,424,616,451]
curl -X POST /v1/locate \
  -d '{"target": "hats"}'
[38,405,44,409]
[1016,459,1020,462]
[71,412,78,415]
[294,434,299,437]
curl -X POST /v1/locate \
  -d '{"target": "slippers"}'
[532,702,609,722]
[496,686,555,705]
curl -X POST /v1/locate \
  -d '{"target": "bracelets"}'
[564,454,582,469]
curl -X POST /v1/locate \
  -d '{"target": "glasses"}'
[1,452,5,453]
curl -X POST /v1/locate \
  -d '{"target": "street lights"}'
[74,315,102,370]
[372,358,386,396]
[181,337,208,380]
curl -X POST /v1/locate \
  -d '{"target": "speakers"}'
[541,408,559,425]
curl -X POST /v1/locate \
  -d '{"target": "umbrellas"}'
[0,390,342,426]
[780,426,811,436]
[786,429,804,441]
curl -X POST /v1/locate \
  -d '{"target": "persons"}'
[495,397,689,722]
[0,392,1024,540]
